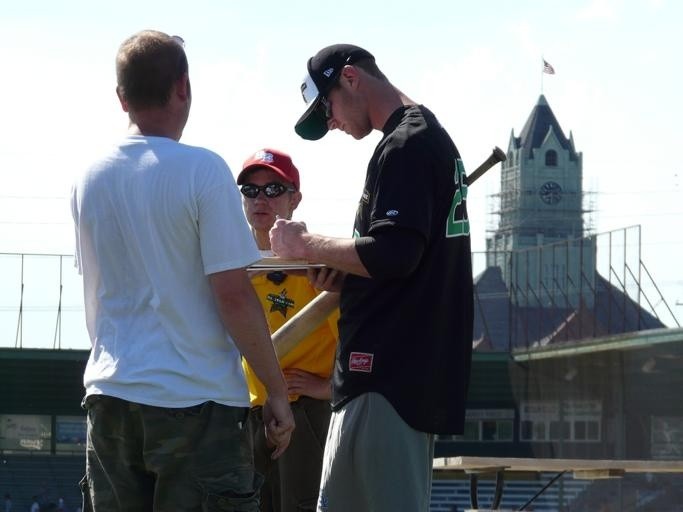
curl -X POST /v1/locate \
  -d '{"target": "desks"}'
[433,455,682,510]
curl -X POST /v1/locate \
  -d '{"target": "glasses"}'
[319,88,334,119]
[173,35,185,47]
[240,181,297,198]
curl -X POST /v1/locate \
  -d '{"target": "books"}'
[246,254,328,271]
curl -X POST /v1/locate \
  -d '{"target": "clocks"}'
[539,181,562,205]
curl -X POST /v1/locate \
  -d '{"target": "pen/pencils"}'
[275,215,280,219]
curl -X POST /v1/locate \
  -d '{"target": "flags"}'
[541,59,555,75]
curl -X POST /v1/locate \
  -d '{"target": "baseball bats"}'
[271,146,505,361]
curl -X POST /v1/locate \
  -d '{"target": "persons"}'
[28,494,41,511]
[265,42,477,511]
[69,29,298,511]
[3,493,13,511]
[233,148,342,512]
[57,494,65,511]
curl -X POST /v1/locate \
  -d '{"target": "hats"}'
[294,44,375,140]
[237,148,300,191]
